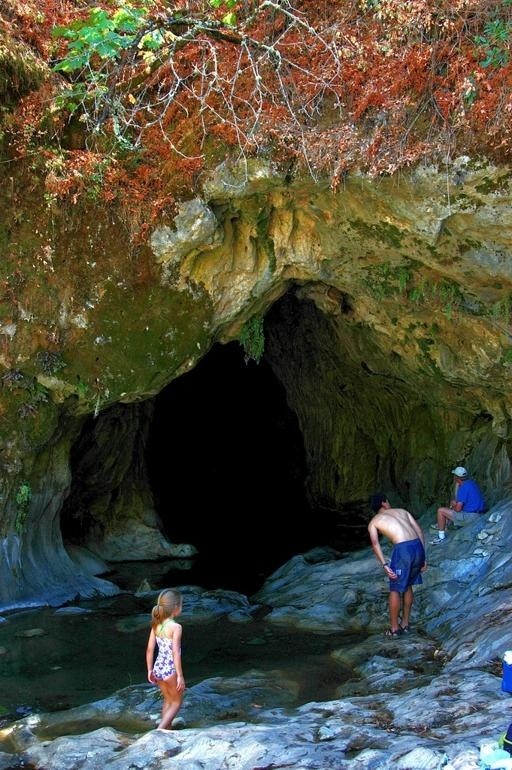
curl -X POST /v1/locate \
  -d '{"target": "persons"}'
[146,590,185,729]
[367,494,428,637]
[428,467,484,545]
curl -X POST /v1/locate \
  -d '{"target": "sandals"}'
[383,624,409,640]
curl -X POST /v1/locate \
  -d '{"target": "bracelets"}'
[383,562,388,568]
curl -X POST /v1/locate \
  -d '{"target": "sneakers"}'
[428,535,449,545]
[430,523,440,530]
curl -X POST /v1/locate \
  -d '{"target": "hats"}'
[451,467,468,478]
